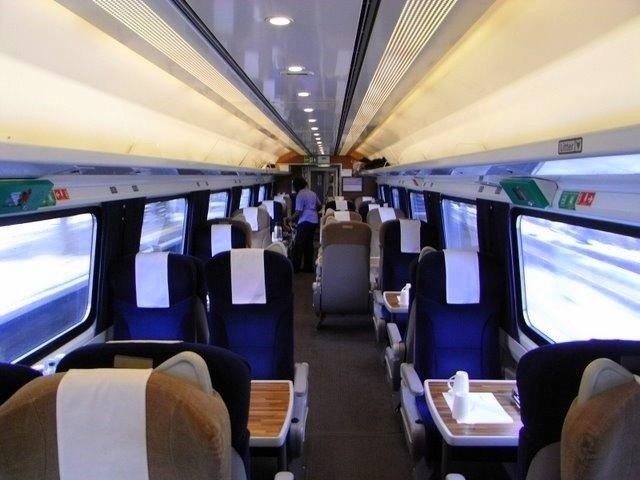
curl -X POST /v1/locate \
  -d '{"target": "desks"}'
[423,379,522,478]
[383,290,409,321]
[248,380,294,472]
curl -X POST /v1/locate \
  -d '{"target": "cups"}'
[399,290,409,306]
[401,283,411,290]
[447,370,470,397]
[451,391,468,420]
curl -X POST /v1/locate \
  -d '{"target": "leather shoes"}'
[300,265,314,272]
[295,268,303,274]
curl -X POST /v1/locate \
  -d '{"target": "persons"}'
[287,176,321,273]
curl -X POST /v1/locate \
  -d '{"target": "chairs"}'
[195,218,251,247]
[203,248,310,459]
[374,220,432,342]
[232,207,271,248]
[56,340,251,454]
[516,339,640,480]
[325,200,354,210]
[355,195,376,211]
[366,207,406,254]
[312,222,371,329]
[259,201,282,229]
[106,252,209,341]
[321,208,363,222]
[0,362,43,405]
[401,250,502,456]
[0,368,232,479]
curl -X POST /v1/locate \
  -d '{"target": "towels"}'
[443,389,515,427]
[396,291,410,307]
